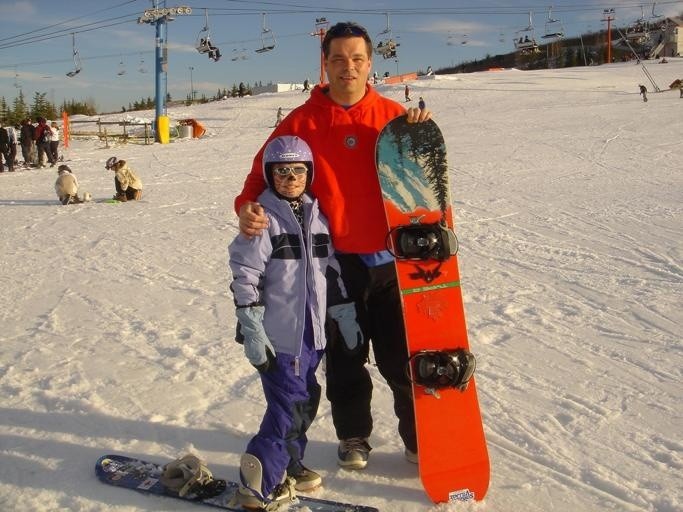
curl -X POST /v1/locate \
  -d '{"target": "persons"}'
[639,84,648,103]
[302,78,310,92]
[54,164,80,205]
[274,106,285,127]
[200,38,215,59]
[232,20,432,471]
[227,134,367,512]
[524,35,532,42]
[519,37,523,42]
[204,36,223,59]
[404,85,411,102]
[0,117,61,173]
[418,96,425,110]
[103,156,142,203]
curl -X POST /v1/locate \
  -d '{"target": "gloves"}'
[235,303,278,374]
[327,298,364,355]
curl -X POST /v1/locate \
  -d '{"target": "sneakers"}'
[63,193,72,205]
[236,476,312,512]
[337,436,373,471]
[287,458,323,490]
[403,447,418,463]
[0,157,60,172]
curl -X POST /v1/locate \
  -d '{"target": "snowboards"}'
[374,113,490,505]
[94,454,378,512]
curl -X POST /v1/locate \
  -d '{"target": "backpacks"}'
[41,127,50,144]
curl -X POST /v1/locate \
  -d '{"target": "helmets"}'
[104,157,120,169]
[261,135,315,190]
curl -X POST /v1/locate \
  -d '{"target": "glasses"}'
[273,166,308,176]
[326,24,364,39]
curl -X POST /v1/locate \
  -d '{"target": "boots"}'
[113,192,129,202]
